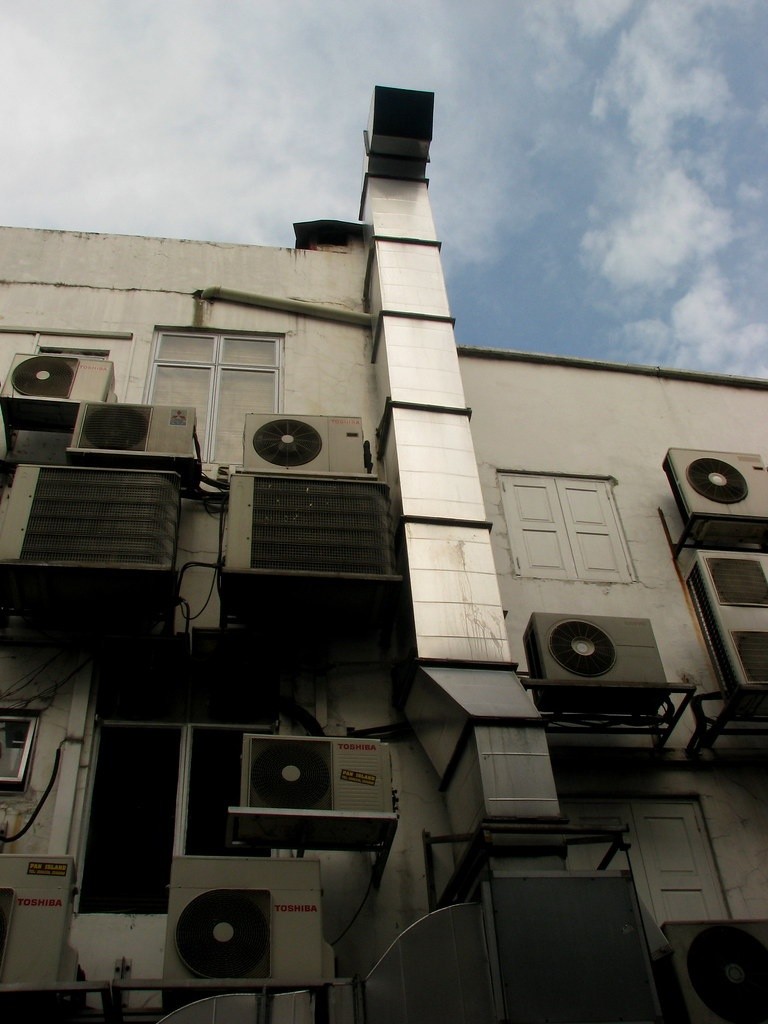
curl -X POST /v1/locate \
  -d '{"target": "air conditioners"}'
[682,550,768,697]
[217,473,404,637]
[0,462,186,622]
[160,854,336,982]
[0,853,80,982]
[663,447,768,543]
[520,613,667,713]
[0,353,115,433]
[243,413,367,474]
[70,401,197,457]
[653,918,768,1024]
[241,732,392,814]
